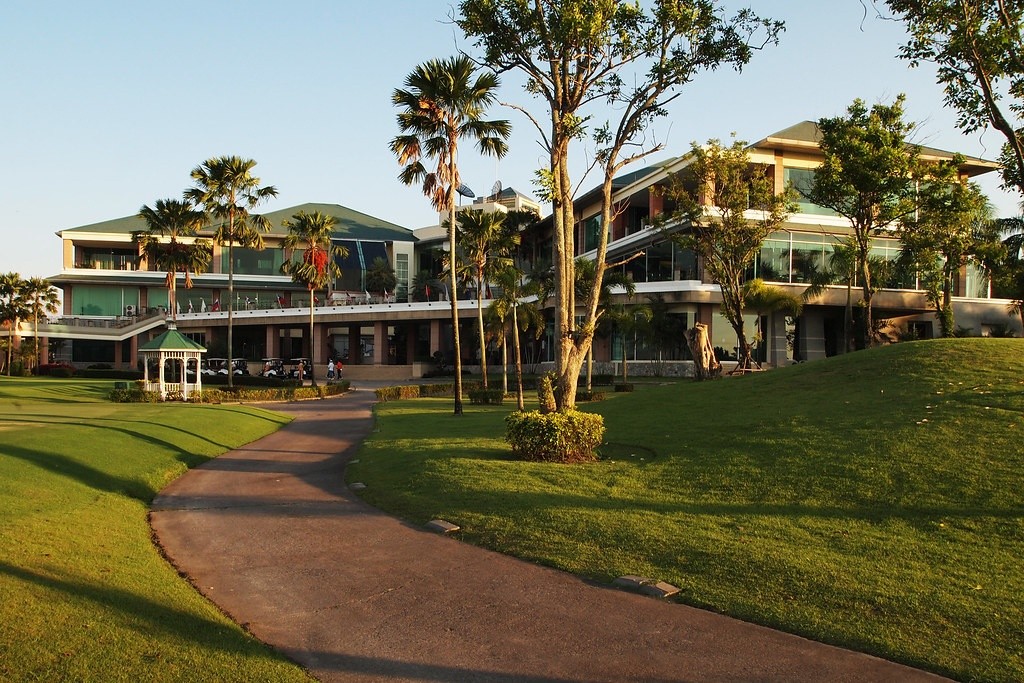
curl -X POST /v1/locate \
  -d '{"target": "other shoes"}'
[328,377,331,379]
[330,378,334,380]
[336,378,339,380]
[340,378,343,380]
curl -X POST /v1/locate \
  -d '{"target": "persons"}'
[298,362,303,380]
[326,358,335,380]
[336,359,342,379]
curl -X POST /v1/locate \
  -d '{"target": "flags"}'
[385,291,389,300]
[212,299,219,312]
[188,300,192,313]
[177,302,180,317]
[346,292,351,300]
[201,300,206,313]
[366,291,371,301]
[278,296,286,305]
[426,287,430,296]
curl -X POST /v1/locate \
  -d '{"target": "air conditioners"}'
[126,304,136,316]
